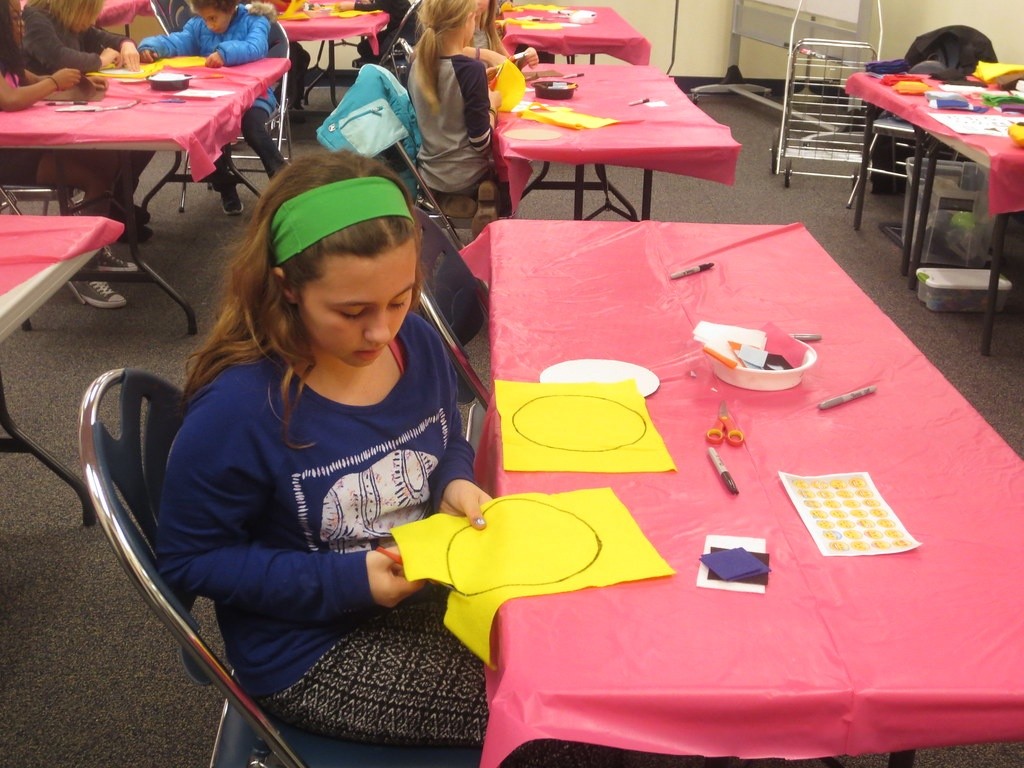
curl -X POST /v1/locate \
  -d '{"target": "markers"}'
[789,332,822,341]
[671,262,716,280]
[819,386,878,410]
[708,448,739,495]
[557,11,571,15]
[562,74,584,78]
[629,99,650,106]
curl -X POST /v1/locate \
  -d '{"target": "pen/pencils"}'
[532,18,555,22]
[101,43,116,63]
[554,15,570,18]
[45,100,87,107]
[158,98,188,103]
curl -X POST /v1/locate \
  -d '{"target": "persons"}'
[139,0,287,212]
[339,0,420,68]
[463,0,539,71]
[0,0,152,308]
[261,0,311,109]
[156,153,620,768]
[403,0,504,236]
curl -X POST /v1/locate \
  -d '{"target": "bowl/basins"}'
[707,338,817,391]
[304,10,331,17]
[569,11,597,24]
[146,74,193,90]
[531,81,578,99]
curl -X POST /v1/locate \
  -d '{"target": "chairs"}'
[179,20,292,215]
[412,205,490,410]
[0,184,88,306]
[314,62,466,250]
[150,0,191,36]
[77,366,482,768]
[846,24,998,210]
[352,0,423,83]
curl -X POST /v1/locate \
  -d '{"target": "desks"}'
[94,0,155,39]
[277,0,391,108]
[491,63,744,222]
[0,54,291,337]
[0,214,127,527]
[456,219,1024,768]
[496,5,653,66]
[843,72,1024,357]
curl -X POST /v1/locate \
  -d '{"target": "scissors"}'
[189,73,226,79]
[490,63,504,91]
[707,401,744,446]
[376,546,456,591]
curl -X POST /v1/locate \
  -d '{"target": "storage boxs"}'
[900,156,997,268]
[916,267,1012,314]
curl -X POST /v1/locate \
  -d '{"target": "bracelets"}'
[476,48,480,60]
[48,77,58,88]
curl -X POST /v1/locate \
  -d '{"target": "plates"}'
[540,359,660,398]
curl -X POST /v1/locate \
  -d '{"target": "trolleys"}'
[771,0,885,191]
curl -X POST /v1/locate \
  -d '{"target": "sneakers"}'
[71,279,126,307]
[82,246,137,272]
[220,184,244,215]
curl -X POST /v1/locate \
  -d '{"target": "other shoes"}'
[472,181,500,240]
[417,190,477,218]
[126,205,151,223]
[118,221,153,244]
